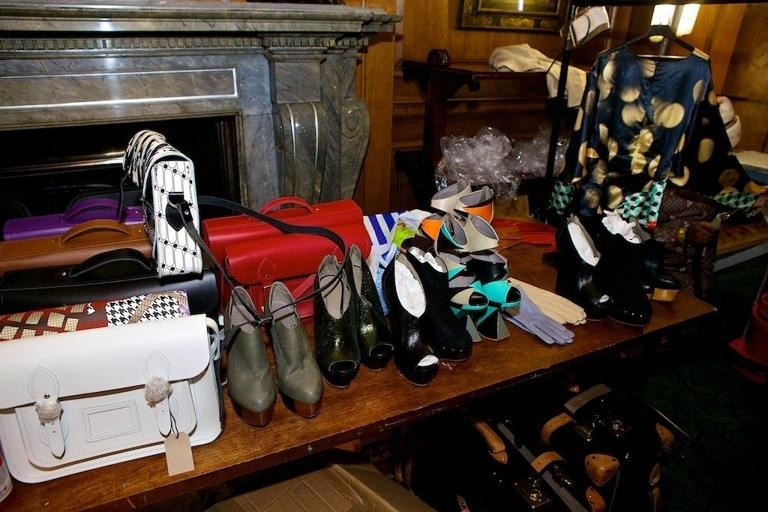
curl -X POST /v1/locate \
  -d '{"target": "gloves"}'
[500,275,587,344]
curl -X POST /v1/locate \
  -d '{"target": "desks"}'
[0,214,718,512]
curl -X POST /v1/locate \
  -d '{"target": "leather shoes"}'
[557,211,679,328]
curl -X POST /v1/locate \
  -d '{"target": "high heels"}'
[224,285,277,428]
[264,281,324,418]
[311,180,521,389]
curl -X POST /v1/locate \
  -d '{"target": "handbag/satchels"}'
[202,198,369,323]
[0,130,226,490]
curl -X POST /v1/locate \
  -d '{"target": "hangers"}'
[625,25,694,62]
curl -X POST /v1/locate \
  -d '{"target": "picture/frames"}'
[458,0,568,35]
